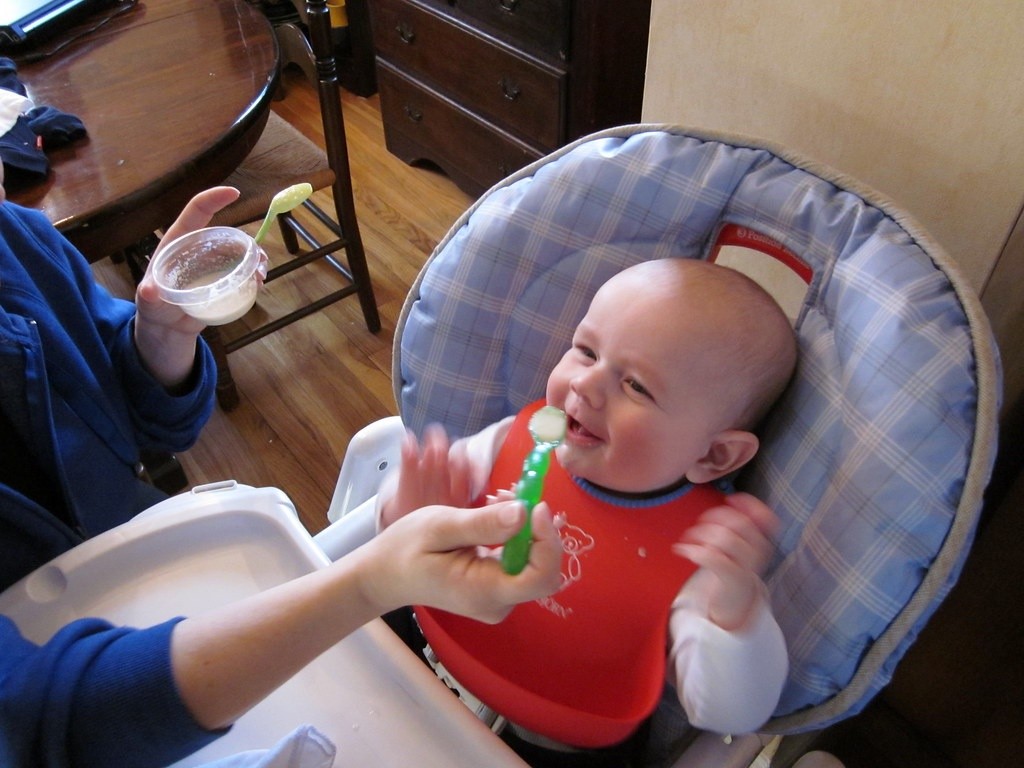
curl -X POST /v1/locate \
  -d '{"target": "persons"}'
[0,151,564,768]
[371,257,799,768]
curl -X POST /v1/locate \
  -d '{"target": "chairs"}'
[0,123,1003,768]
[123,0,382,413]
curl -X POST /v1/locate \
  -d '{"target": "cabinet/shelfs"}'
[372,0,652,199]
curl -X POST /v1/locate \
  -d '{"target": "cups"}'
[150,226,260,327]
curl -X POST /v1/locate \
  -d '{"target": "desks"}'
[5,0,282,265]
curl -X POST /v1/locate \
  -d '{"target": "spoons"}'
[252,182,312,245]
[502,405,569,575]
